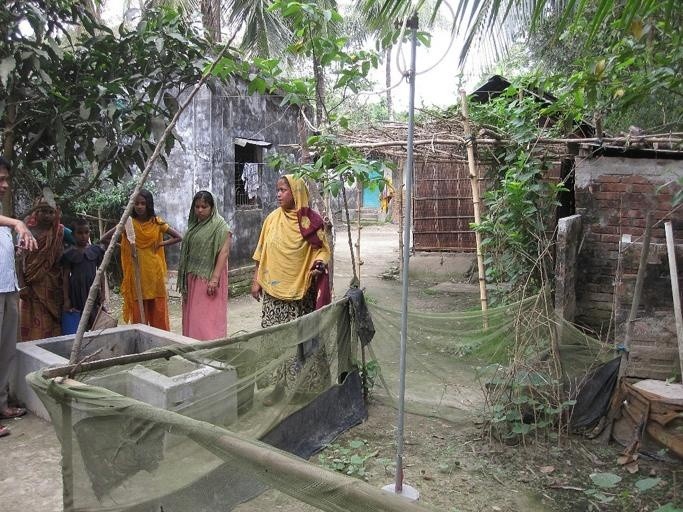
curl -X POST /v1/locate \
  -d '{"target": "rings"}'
[28,235,33,242]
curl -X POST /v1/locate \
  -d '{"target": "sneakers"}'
[0,424,10,437]
[0,407,27,418]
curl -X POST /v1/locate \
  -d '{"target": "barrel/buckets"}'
[210,329,259,417]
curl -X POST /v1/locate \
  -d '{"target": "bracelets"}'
[210,280,218,284]
[251,279,257,283]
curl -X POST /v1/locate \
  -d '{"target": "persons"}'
[249,174,331,407]
[174,190,234,342]
[13,196,65,342]
[99,188,183,332]
[0,156,38,437]
[52,219,106,332]
